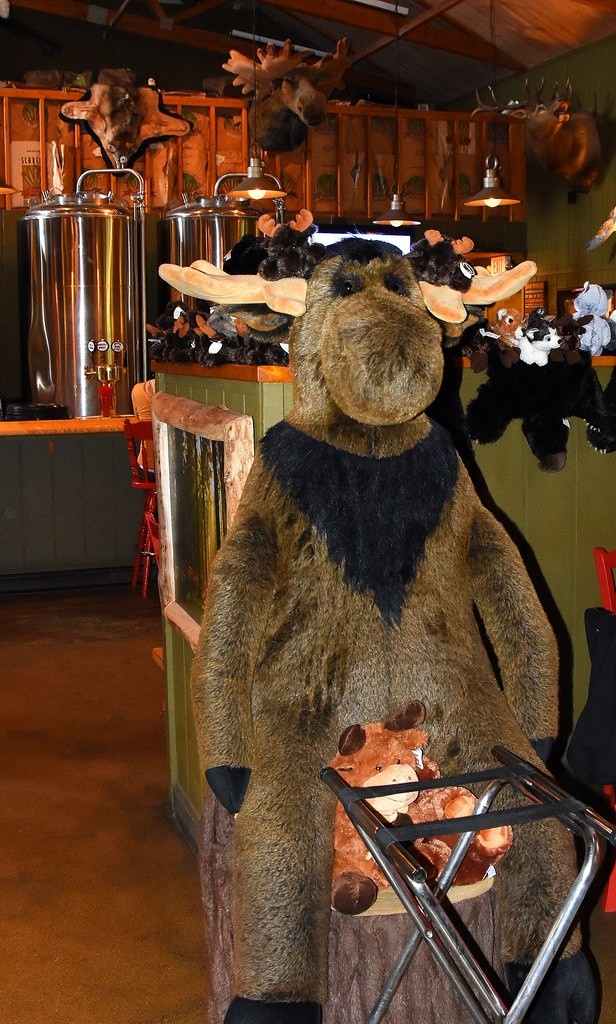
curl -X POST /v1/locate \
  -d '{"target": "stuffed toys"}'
[407,230,474,291]
[466,281,616,472]
[255,210,319,281]
[471,76,601,185]
[221,38,351,153]
[330,699,514,916]
[145,233,289,369]
[158,239,605,1024]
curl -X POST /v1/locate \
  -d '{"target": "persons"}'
[131,379,155,481]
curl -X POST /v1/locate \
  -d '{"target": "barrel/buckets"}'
[20,169,146,414]
[156,172,285,309]
